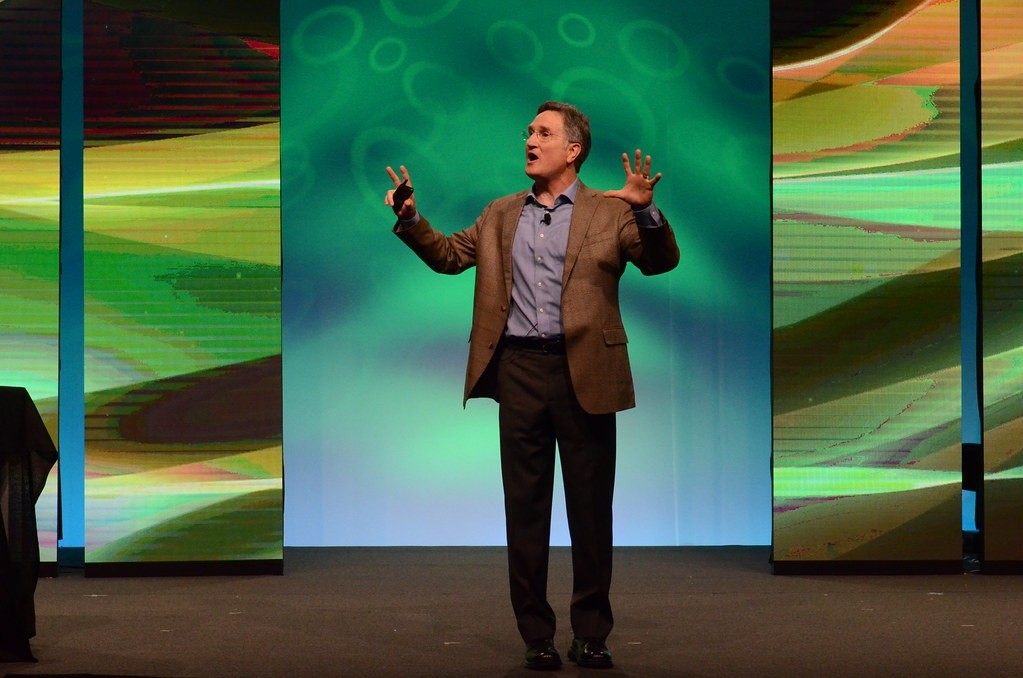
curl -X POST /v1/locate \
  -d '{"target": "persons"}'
[383,100,681,670]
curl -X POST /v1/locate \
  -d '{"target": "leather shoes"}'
[567,638,614,668]
[523,637,564,669]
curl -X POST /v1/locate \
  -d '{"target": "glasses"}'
[520,128,567,143]
